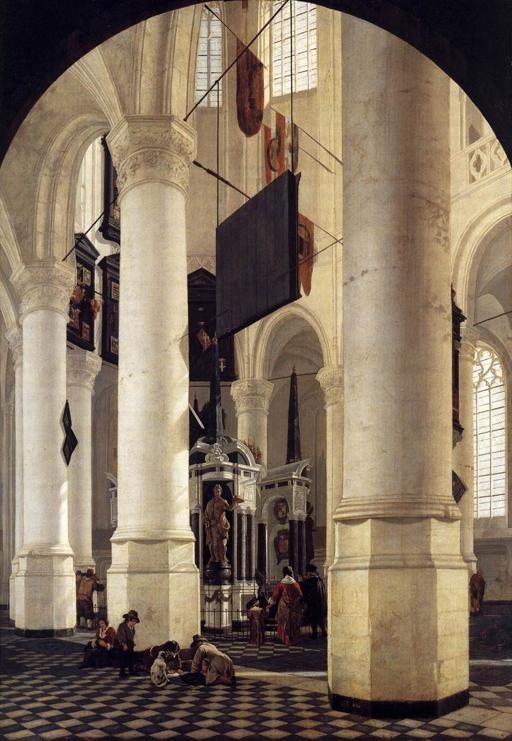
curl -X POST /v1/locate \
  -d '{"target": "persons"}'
[76,481,327,689]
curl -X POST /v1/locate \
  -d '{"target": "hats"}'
[77,568,96,577]
[191,635,207,647]
[122,611,140,624]
